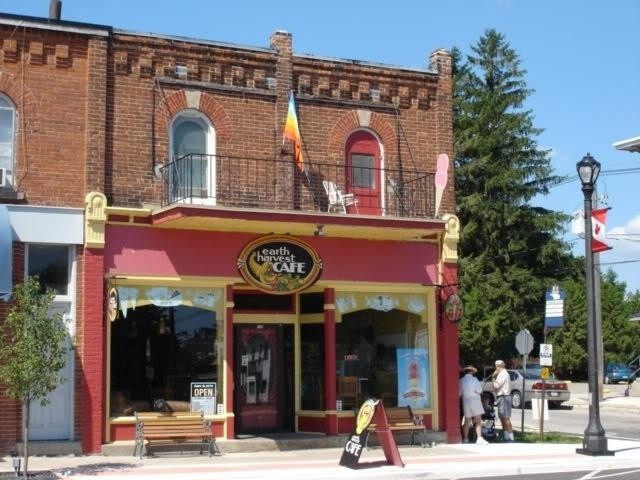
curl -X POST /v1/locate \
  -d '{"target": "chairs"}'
[337,376,359,408]
[323,181,359,214]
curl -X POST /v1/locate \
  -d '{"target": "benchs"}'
[133,411,213,460]
[352,405,426,448]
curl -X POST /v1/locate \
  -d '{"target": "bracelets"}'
[491,376,494,379]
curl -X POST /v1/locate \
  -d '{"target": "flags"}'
[283,91,309,185]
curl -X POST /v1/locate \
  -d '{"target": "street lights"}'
[572,148,618,459]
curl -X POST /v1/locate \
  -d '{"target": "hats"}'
[495,360,504,366]
[463,365,478,374]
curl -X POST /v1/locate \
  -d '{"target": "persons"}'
[459,365,489,444]
[482,393,495,418]
[490,359,516,442]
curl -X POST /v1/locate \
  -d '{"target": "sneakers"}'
[463,436,488,445]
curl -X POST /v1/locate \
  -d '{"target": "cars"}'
[575,352,636,389]
[517,361,559,385]
[476,368,572,410]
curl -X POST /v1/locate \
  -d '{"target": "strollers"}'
[463,390,501,442]
